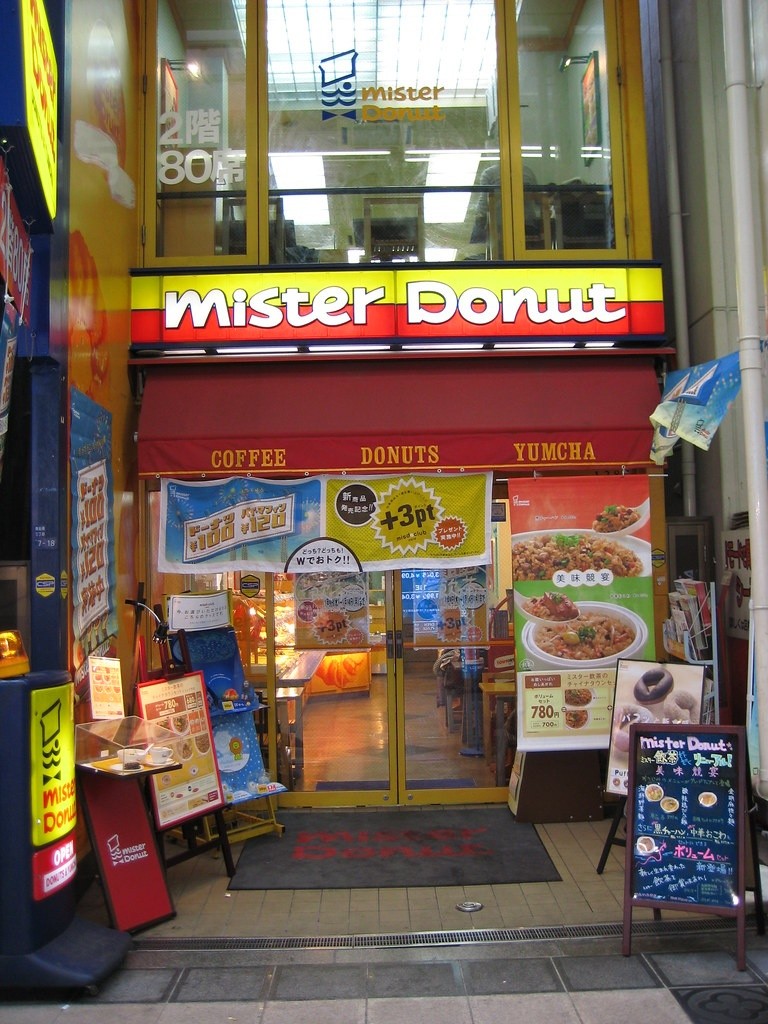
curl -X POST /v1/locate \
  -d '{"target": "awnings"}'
[137,368,669,480]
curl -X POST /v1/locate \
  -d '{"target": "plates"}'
[565,689,597,710]
[110,764,143,772]
[146,757,175,766]
[512,529,651,576]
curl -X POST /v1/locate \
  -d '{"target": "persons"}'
[473,165,541,225]
[502,709,518,769]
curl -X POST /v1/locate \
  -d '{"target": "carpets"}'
[226,808,564,891]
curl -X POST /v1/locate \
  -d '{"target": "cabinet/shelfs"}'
[209,702,288,805]
[368,589,386,633]
[663,578,719,725]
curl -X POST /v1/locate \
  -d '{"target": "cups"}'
[149,747,173,763]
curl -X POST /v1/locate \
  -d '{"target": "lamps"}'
[167,59,202,80]
[558,53,592,73]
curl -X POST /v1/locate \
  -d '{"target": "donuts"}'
[609,667,698,751]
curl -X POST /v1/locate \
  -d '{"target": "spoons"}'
[592,497,649,538]
[514,589,581,626]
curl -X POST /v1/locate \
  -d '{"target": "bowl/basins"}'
[521,600,648,670]
[117,749,146,762]
[563,709,593,731]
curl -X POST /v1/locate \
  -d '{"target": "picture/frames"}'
[580,51,602,167]
[161,58,178,151]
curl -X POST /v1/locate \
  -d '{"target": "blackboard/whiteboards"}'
[623,724,760,915]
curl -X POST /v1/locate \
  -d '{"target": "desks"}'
[478,682,516,766]
[255,686,306,792]
[452,661,486,742]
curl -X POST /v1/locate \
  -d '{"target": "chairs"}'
[363,197,426,262]
[485,191,552,260]
[554,191,616,249]
[222,198,284,265]
